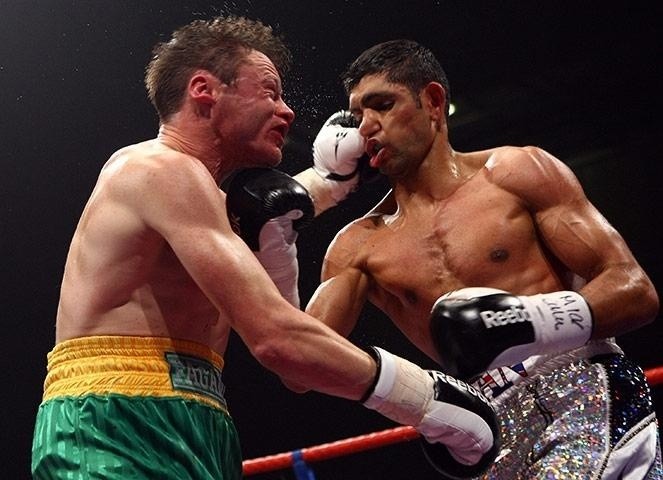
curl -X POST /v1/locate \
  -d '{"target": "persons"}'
[31,11,503,479]
[221,33,662,479]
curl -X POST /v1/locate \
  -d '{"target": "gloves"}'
[291,111,366,216]
[227,166,315,308]
[357,346,501,479]
[429,287,593,383]
